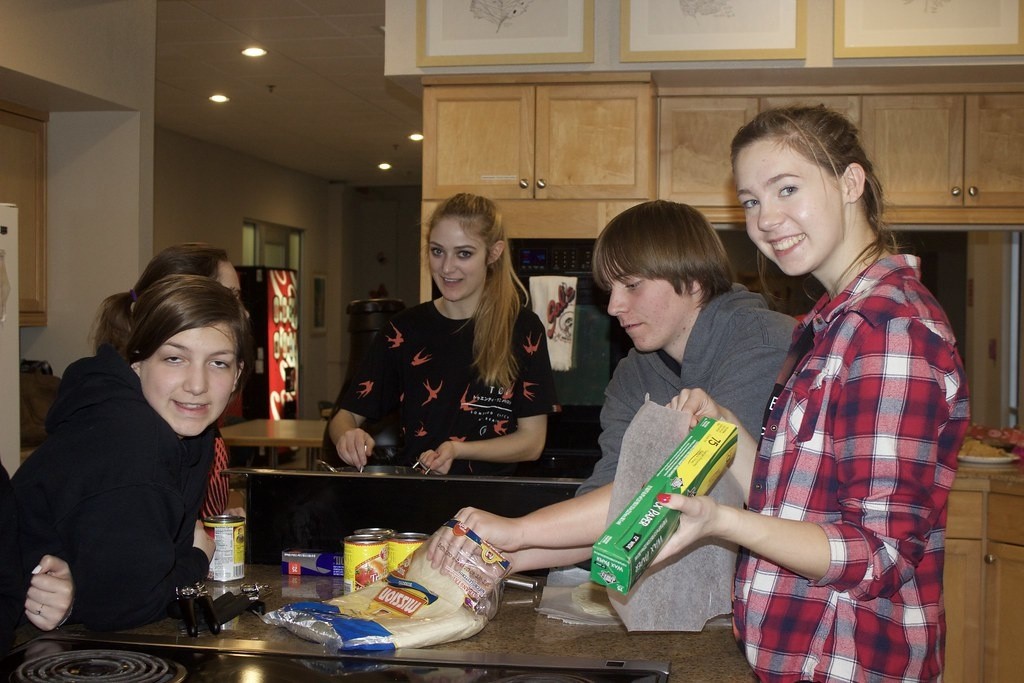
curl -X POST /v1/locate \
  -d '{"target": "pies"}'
[571,580,619,616]
[292,554,488,649]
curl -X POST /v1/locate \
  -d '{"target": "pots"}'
[315,459,430,476]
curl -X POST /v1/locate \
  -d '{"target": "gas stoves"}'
[0,630,672,683]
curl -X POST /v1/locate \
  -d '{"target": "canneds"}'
[202,515,246,582]
[343,526,430,595]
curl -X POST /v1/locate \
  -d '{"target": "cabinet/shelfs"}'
[0,107,48,327]
[424,75,657,222]
[660,83,860,226]
[942,491,986,683]
[867,79,1024,226]
[985,491,1024,683]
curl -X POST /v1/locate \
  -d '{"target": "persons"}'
[326,192,557,476]
[426,200,798,595]
[0,274,252,656]
[647,102,970,683]
[97,243,246,517]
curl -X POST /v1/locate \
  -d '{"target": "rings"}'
[37,604,44,615]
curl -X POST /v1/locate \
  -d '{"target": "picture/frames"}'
[833,0,1024,57]
[416,0,595,68]
[311,274,328,335]
[619,1,808,65]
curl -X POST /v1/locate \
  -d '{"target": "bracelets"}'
[57,608,72,628]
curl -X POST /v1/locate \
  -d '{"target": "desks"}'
[220,419,327,467]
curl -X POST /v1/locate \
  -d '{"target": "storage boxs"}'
[593,416,739,597]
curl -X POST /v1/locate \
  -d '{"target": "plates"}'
[957,454,1021,464]
